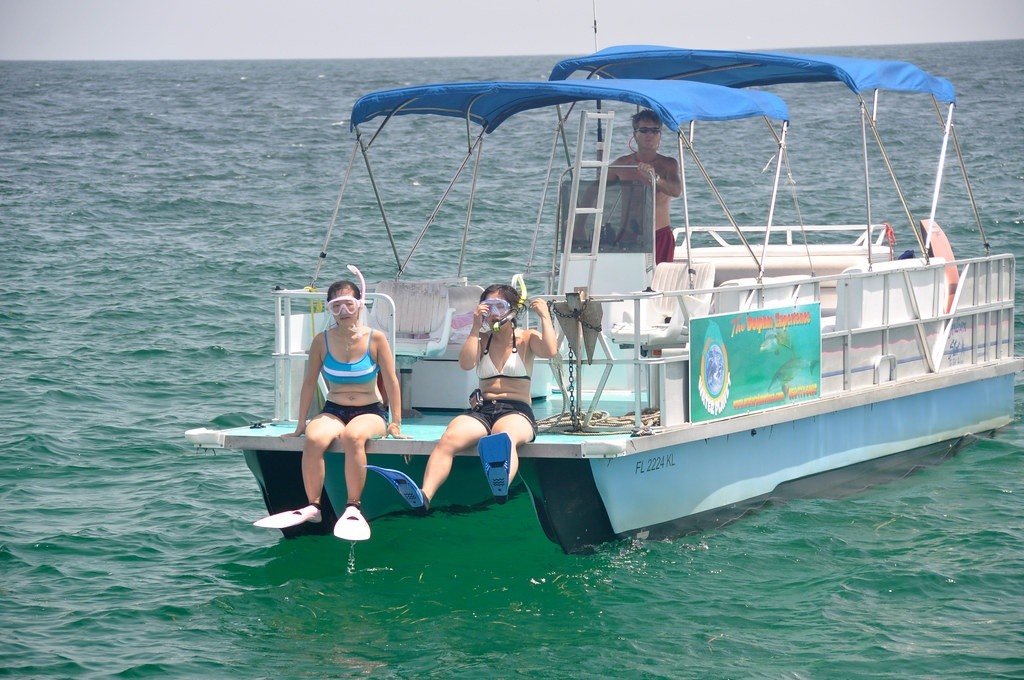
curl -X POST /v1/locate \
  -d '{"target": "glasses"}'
[635,127,661,134]
[326,296,360,315]
[480,298,512,318]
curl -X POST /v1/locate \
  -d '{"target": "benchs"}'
[714,275,819,314]
[821,257,949,333]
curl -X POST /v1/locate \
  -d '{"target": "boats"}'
[181,44,1024,556]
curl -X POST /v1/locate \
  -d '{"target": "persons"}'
[252,281,414,540]
[363,283,557,514]
[576,109,682,264]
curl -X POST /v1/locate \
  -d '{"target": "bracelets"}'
[394,424,400,429]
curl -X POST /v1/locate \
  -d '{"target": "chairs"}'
[370,281,452,419]
[449,285,485,311]
[610,262,716,409]
